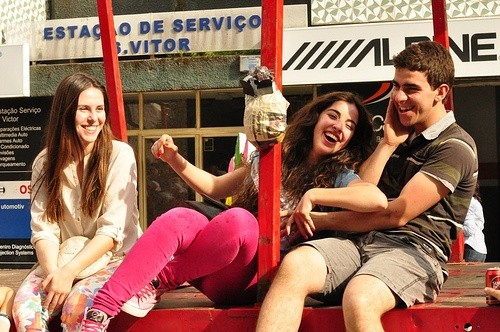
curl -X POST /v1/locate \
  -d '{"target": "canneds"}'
[486,266,500,305]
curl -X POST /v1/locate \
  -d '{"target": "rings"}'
[302,221,306,225]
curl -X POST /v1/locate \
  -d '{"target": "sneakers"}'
[80,307,114,331]
[120,279,162,318]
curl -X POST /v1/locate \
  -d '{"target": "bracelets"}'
[0,314,11,323]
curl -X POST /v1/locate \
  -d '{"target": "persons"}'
[463,183,487,263]
[255,42,478,332]
[13,72,140,332]
[0,287,15,332]
[79,93,388,332]
